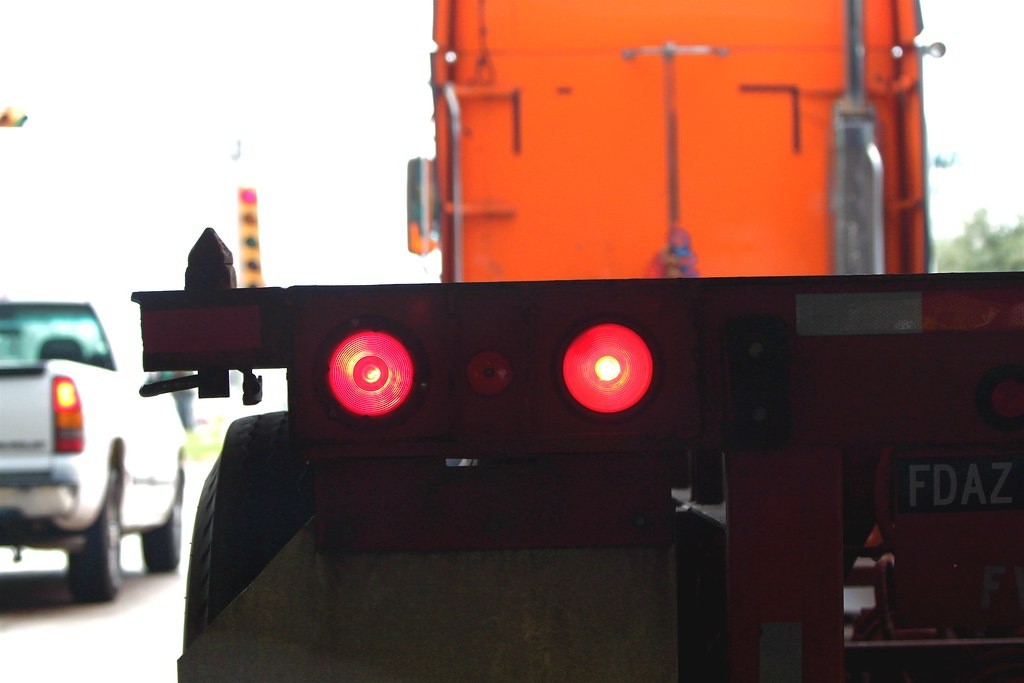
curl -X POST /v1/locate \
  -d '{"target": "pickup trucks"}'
[0,298,188,602]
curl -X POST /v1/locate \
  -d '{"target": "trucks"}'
[131,0,1024,683]
